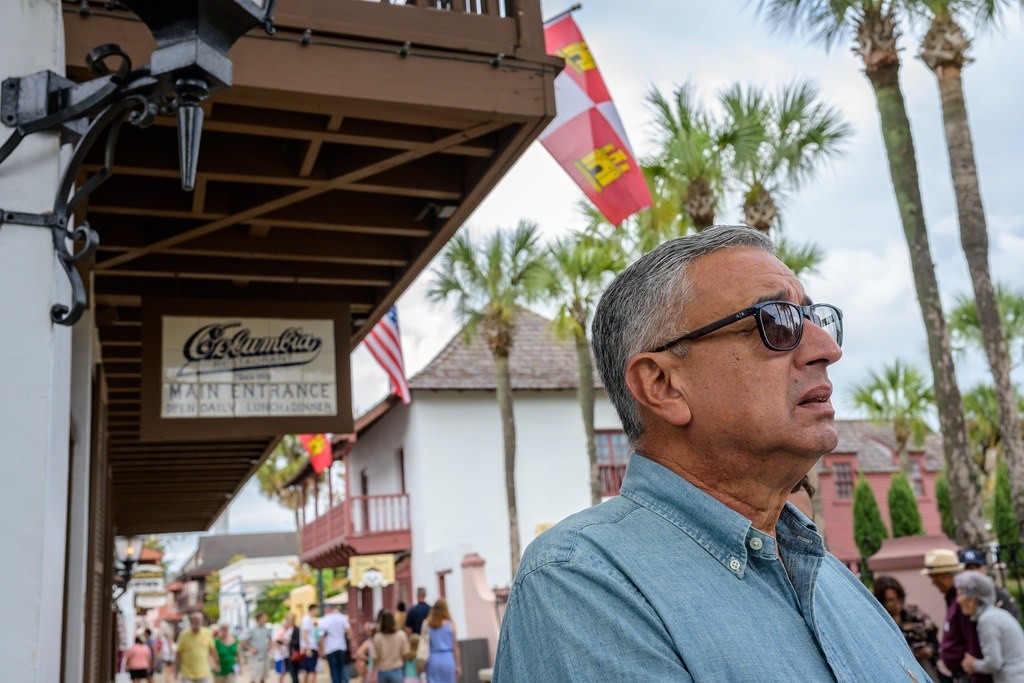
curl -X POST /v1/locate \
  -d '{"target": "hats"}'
[919,550,964,576]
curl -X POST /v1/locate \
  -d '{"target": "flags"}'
[363,306,411,404]
[537,14,651,225]
[300,434,333,473]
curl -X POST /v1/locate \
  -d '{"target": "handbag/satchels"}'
[416,622,431,675]
[291,651,303,661]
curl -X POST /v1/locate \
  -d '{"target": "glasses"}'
[653,303,844,352]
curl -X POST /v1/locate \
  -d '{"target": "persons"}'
[492,225,934,683]
[125,623,178,683]
[354,588,463,683]
[175,612,220,683]
[237,604,355,683]
[872,549,1024,683]
[211,624,243,683]
